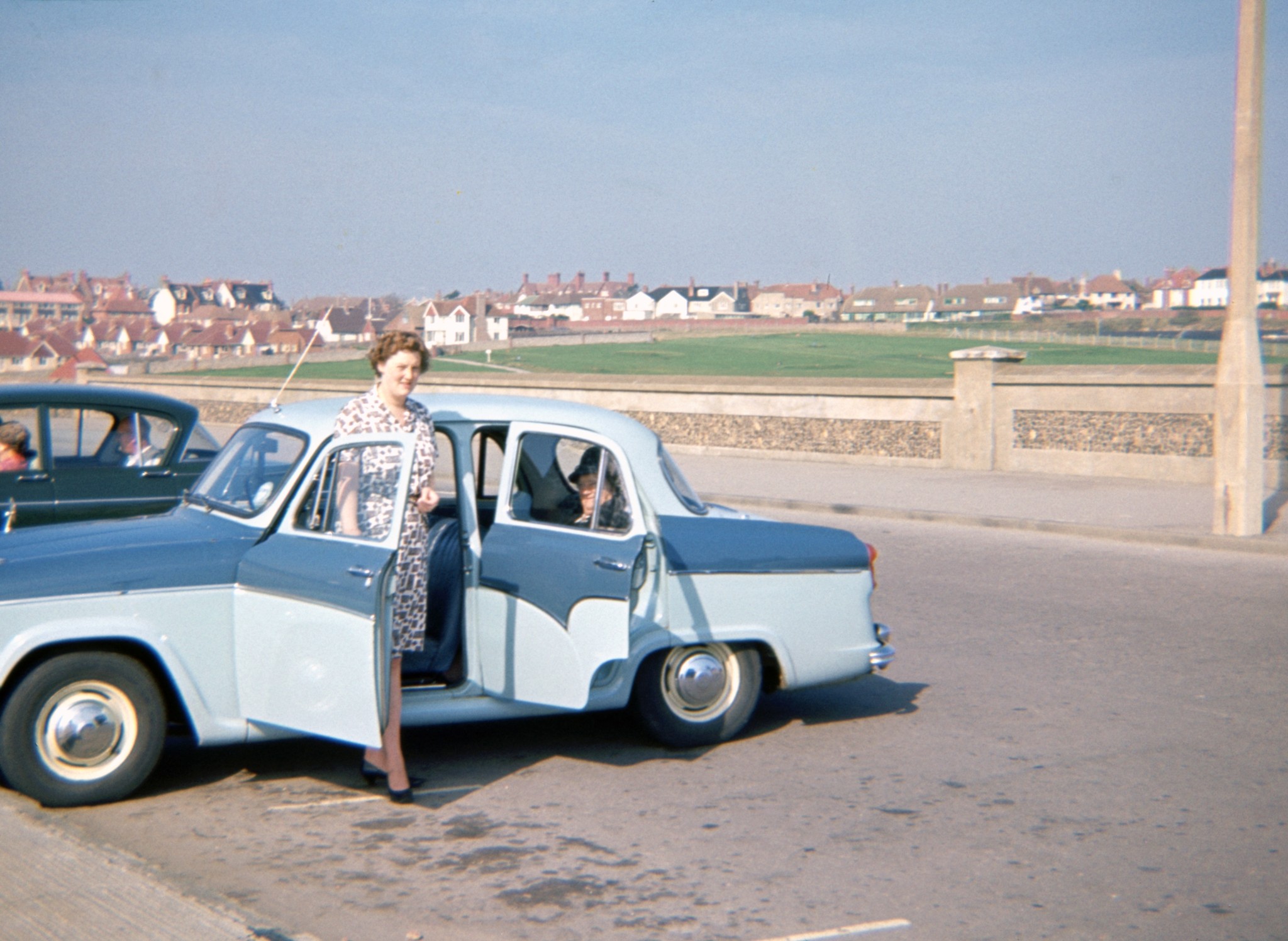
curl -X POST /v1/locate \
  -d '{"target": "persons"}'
[0,421,30,470]
[115,416,163,465]
[557,447,632,529]
[334,333,441,803]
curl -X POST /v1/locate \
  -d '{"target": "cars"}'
[0,384,894,814]
[2,381,308,531]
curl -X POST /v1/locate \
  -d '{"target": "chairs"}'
[399,514,463,672]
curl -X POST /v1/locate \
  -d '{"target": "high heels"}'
[362,761,424,788]
[386,777,415,803]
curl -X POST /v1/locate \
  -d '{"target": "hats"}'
[568,446,617,484]
